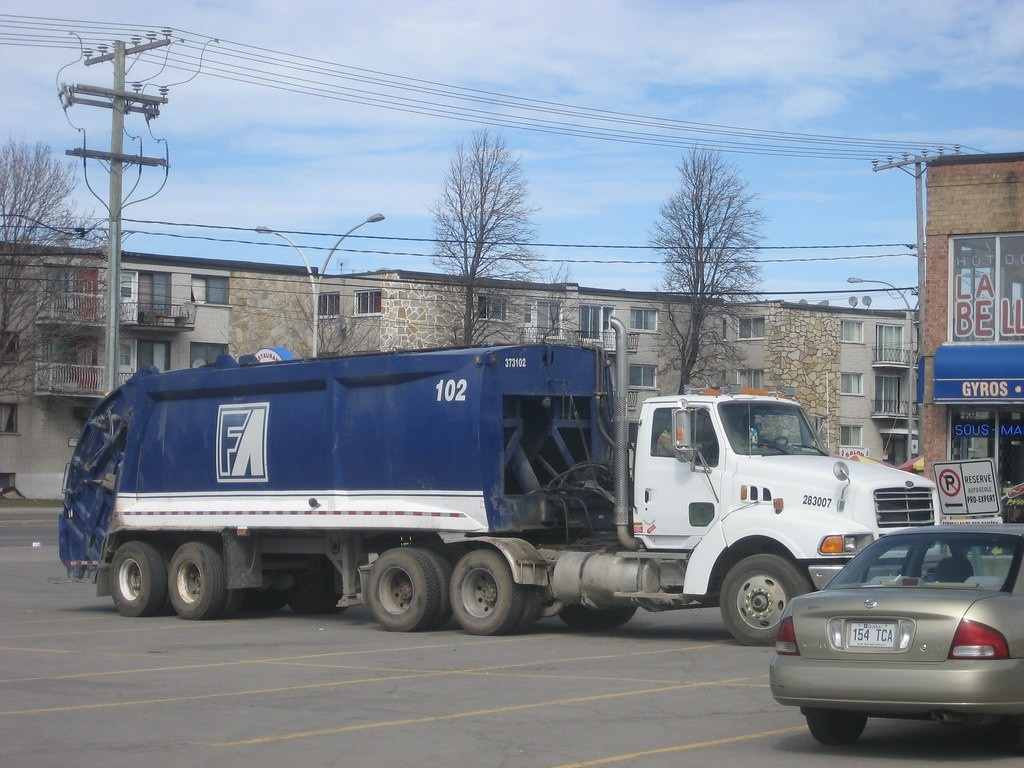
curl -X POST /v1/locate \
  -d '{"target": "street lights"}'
[847,277,912,460]
[254,213,385,358]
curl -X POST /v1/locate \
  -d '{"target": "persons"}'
[733,413,765,450]
[657,412,693,462]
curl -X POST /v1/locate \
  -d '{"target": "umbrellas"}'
[830,454,924,473]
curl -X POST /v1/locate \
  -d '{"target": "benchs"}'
[871,575,1005,591]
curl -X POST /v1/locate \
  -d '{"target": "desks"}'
[156,315,188,327]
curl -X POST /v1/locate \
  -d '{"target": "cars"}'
[771,522,1024,745]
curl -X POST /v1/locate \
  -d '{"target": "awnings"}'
[933,344,1024,404]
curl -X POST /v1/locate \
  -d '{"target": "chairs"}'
[934,558,974,584]
[137,312,155,326]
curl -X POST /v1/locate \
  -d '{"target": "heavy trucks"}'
[59,314,947,646]
